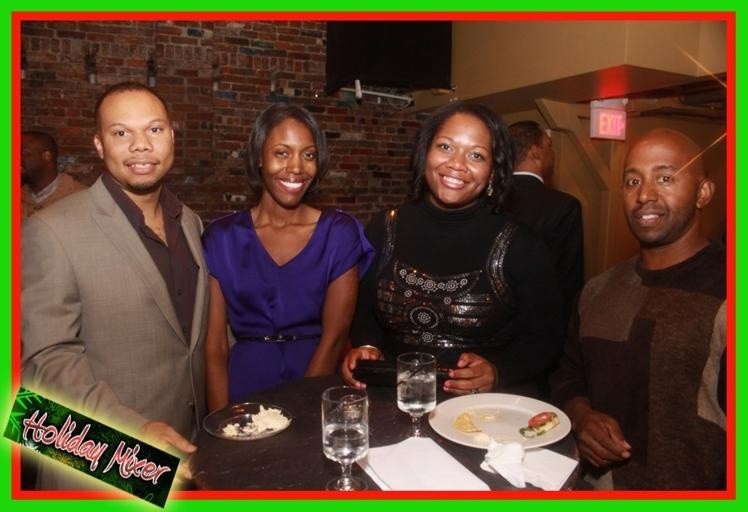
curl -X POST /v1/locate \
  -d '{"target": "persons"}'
[21,130,91,223]
[202,102,376,414]
[553,125,726,490]
[22,81,237,490]
[500,120,584,325]
[341,101,556,396]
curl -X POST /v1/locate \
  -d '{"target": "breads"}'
[520,410,559,438]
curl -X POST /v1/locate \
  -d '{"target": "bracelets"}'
[359,343,379,350]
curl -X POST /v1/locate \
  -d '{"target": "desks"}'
[190,374,580,491]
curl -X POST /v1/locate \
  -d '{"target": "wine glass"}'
[319,386,369,491]
[396,351,439,446]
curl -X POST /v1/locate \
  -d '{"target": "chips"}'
[454,413,482,433]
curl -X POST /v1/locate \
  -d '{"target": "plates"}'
[203,401,296,442]
[427,391,573,451]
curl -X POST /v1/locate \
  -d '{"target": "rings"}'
[471,390,479,394]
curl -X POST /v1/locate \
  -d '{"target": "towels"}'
[355,436,490,491]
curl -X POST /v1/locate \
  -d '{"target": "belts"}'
[233,331,323,347]
